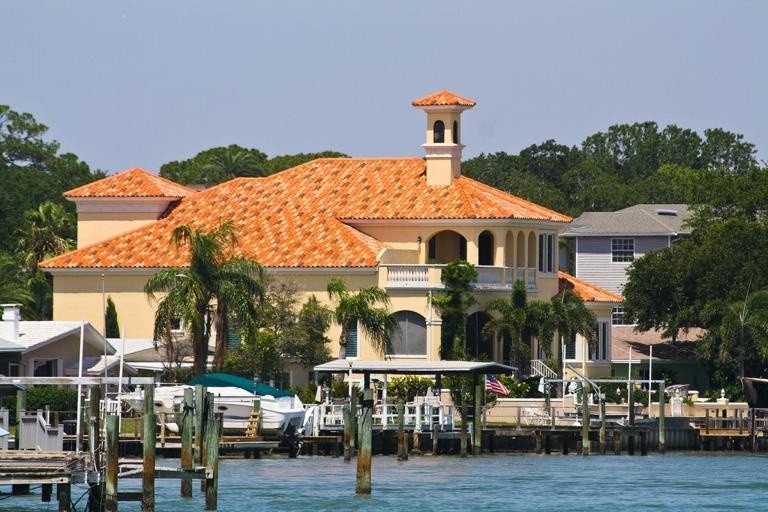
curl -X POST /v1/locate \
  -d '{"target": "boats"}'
[117,381,305,437]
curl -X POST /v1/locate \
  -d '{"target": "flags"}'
[485,374,509,395]
